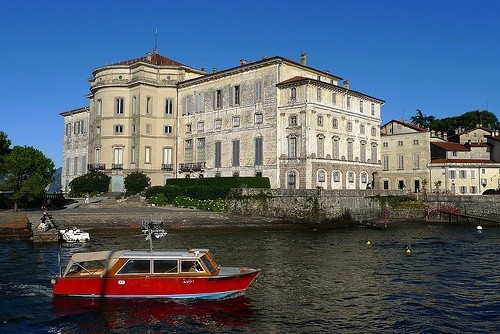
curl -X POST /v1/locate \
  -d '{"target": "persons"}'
[41,214,46,231]
[189,264,198,272]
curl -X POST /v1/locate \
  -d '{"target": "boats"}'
[50,246,262,301]
[141,220,168,239]
[59,226,91,243]
